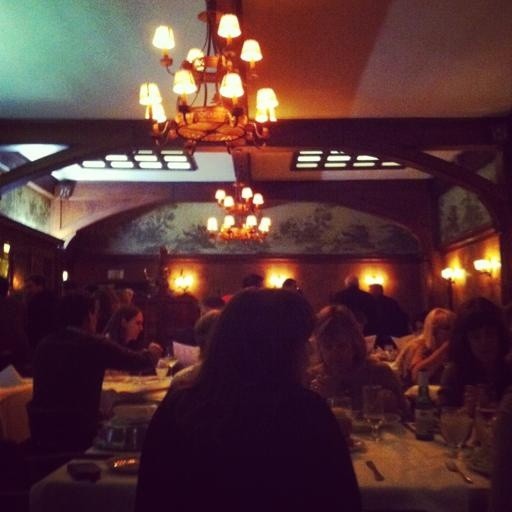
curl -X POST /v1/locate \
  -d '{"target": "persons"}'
[136,287,361,512]
[0,275,512,470]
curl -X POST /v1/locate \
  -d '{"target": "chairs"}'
[0,376,32,443]
[30,370,496,512]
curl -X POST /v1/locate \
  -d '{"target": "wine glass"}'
[441,406,473,458]
[327,396,355,452]
[362,385,385,441]
[155,360,169,384]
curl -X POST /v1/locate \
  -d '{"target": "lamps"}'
[206,155,272,242]
[139,0,280,154]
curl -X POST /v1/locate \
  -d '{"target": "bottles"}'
[413,367,437,441]
